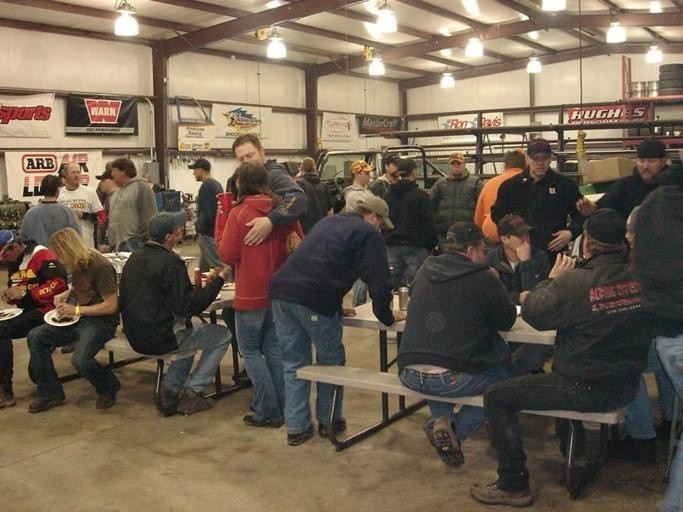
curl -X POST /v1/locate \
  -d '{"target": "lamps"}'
[266,25,286,59]
[526,48,542,73]
[645,38,663,64]
[265,0,282,9]
[465,25,484,59]
[113,1,139,36]
[542,1,568,12]
[606,8,626,44]
[649,1,663,14]
[368,49,385,76]
[440,65,455,90]
[376,1,397,33]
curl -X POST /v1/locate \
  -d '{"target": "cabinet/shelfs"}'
[621,54,683,154]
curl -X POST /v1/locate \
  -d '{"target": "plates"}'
[44,309,81,328]
[1,308,24,322]
[221,283,236,290]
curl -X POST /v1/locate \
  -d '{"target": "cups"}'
[216,191,232,216]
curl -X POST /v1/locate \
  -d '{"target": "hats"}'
[302,157,314,172]
[358,196,394,230]
[349,159,376,175]
[636,138,665,159]
[446,221,485,242]
[381,152,402,163]
[525,139,551,156]
[496,214,536,236]
[448,153,465,165]
[0,230,15,247]
[147,210,186,241]
[586,208,626,244]
[397,159,417,174]
[94,171,113,181]
[187,160,210,171]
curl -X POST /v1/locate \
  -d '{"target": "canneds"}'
[398,287,410,311]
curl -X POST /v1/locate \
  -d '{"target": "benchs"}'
[27,325,197,417]
[296,362,631,500]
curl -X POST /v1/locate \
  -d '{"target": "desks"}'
[189,275,252,402]
[339,292,556,422]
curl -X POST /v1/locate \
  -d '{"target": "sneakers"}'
[28,388,65,413]
[231,370,251,384]
[604,438,656,465]
[94,380,120,410]
[0,392,15,408]
[469,480,532,507]
[159,385,214,417]
[651,419,681,439]
[422,417,464,467]
[318,417,345,437]
[287,426,313,446]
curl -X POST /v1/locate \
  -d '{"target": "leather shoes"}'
[242,401,285,428]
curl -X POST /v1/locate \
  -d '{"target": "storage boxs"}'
[580,154,635,185]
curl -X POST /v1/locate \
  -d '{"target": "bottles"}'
[398,286,410,309]
[193,266,202,286]
